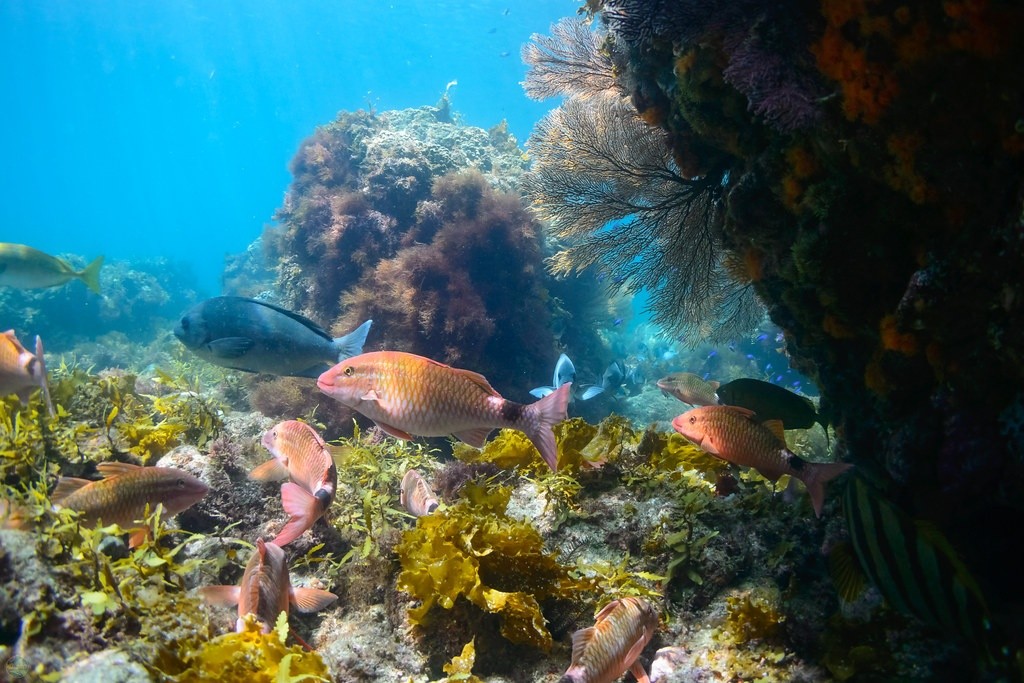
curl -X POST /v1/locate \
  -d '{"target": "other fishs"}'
[1,241,1023,683]
